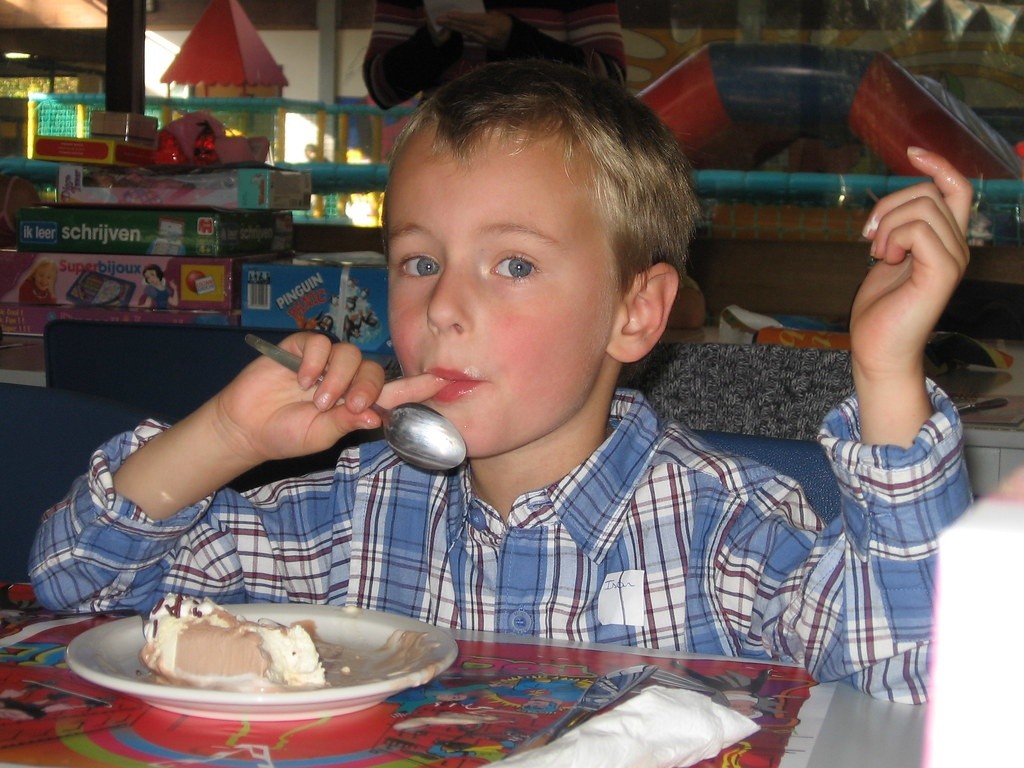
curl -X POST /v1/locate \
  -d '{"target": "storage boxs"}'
[922,469,1024,768]
[0,109,393,353]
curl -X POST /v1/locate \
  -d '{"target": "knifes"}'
[500,663,660,760]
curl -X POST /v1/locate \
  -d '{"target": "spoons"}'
[242,330,469,477]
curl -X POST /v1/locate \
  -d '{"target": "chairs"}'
[1,385,174,583]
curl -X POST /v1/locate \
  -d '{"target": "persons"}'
[18,258,59,305]
[27,60,976,706]
[362,0,627,111]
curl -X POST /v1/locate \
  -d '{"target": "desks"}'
[0,608,920,768]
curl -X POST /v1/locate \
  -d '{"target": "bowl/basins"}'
[64,601,460,724]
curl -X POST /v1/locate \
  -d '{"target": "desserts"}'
[142,591,324,689]
